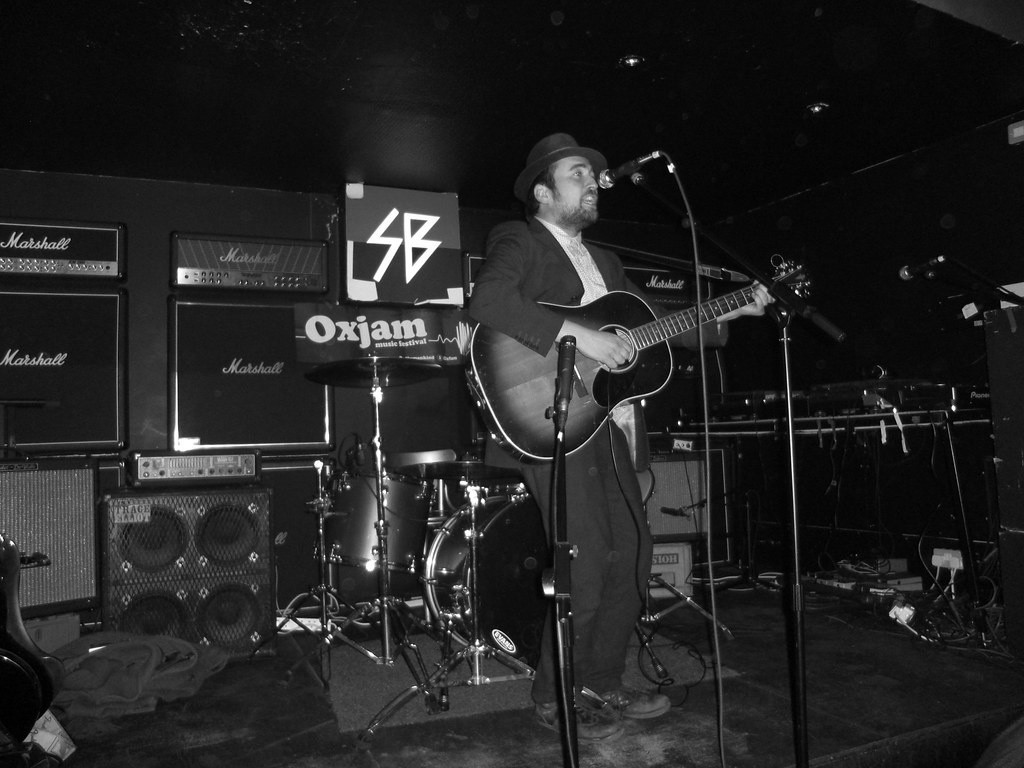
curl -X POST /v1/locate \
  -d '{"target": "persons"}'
[472,134,777,745]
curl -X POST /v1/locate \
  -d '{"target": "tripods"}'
[635,574,737,679]
[246,375,541,742]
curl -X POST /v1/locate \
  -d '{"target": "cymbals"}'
[305,353,446,391]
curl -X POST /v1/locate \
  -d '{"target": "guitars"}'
[461,253,813,465]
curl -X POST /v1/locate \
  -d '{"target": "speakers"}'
[0,285,338,662]
[636,453,712,539]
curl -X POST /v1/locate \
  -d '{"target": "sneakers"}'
[533,684,671,743]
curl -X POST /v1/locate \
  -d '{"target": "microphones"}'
[553,335,577,430]
[900,256,949,279]
[601,150,661,189]
[660,506,688,516]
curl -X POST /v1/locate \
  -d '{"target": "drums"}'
[319,469,436,574]
[420,483,555,651]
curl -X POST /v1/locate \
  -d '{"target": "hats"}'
[513,133,609,206]
